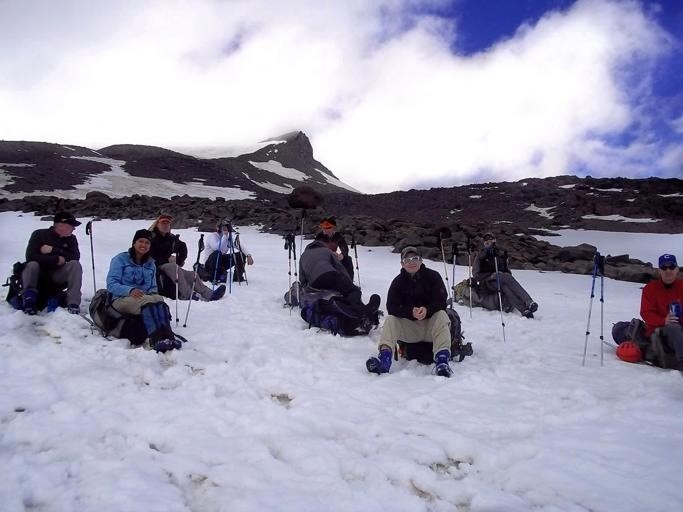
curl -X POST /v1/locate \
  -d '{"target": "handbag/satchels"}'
[479,255,512,273]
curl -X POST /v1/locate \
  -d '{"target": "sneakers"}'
[522,310,533,318]
[67,304,79,314]
[367,294,380,312]
[432,349,454,378]
[210,285,226,301]
[150,339,182,350]
[528,302,537,312]
[22,306,36,315]
[366,351,392,375]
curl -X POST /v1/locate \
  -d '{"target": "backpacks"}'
[300,296,380,336]
[5,261,66,312]
[89,289,147,346]
[612,318,676,369]
[192,262,228,283]
[284,282,343,309]
[397,308,473,365]
[462,278,514,313]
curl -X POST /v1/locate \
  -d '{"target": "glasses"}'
[660,265,676,270]
[402,256,419,264]
[483,236,492,241]
[222,224,229,227]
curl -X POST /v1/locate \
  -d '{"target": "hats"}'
[158,217,171,224]
[133,229,151,244]
[483,232,495,238]
[659,254,676,268]
[401,246,421,262]
[53,211,80,226]
[216,218,231,225]
[319,220,336,228]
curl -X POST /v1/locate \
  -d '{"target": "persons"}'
[366,245,452,378]
[472,233,538,319]
[203,220,253,284]
[106,229,181,353]
[149,215,226,302]
[315,219,354,285]
[21,212,83,314]
[299,242,380,329]
[639,254,683,374]
